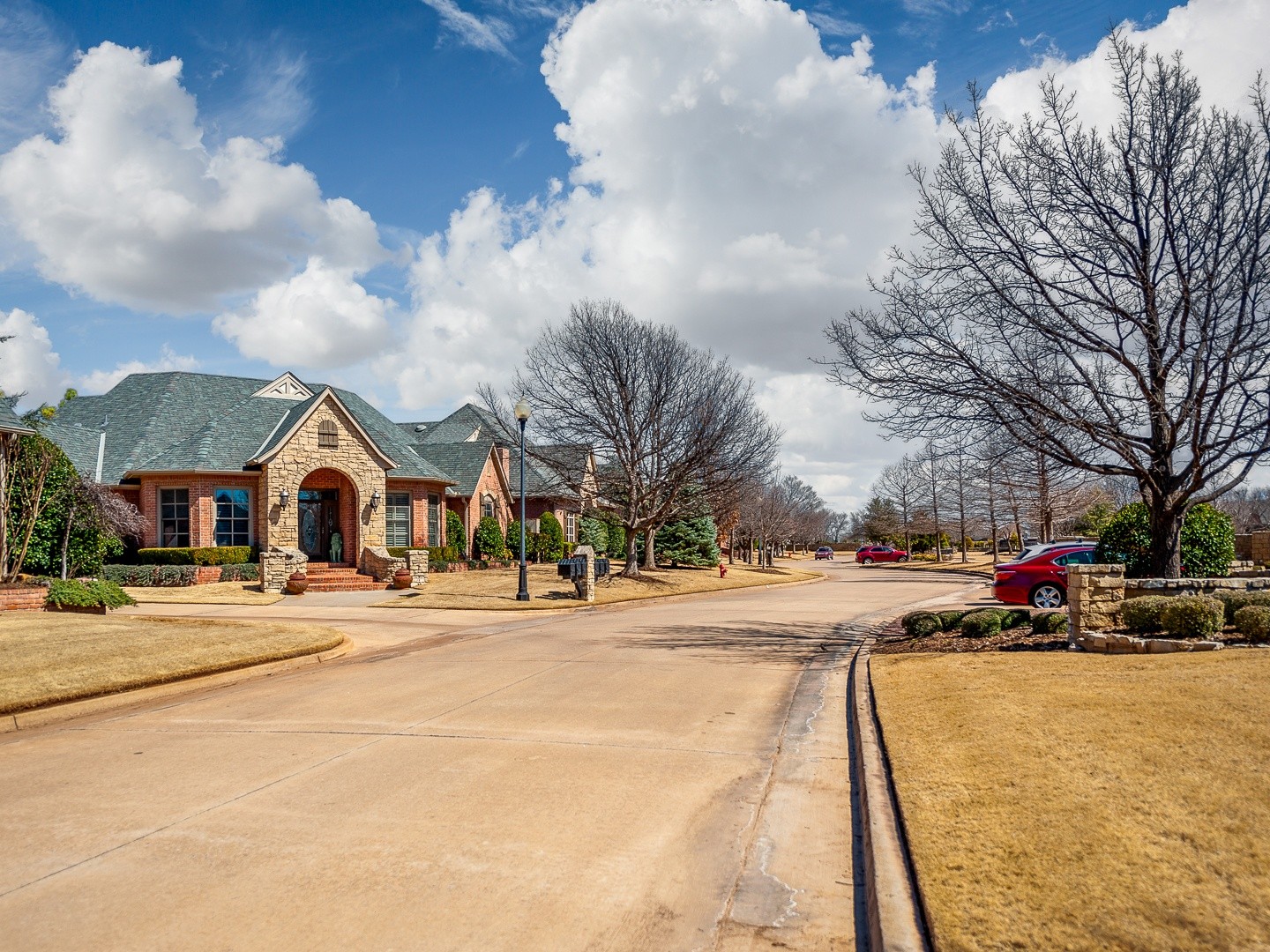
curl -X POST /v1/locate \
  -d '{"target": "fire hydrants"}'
[718,563,727,578]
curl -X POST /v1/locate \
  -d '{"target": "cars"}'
[1010,537,1128,564]
[992,547,1186,608]
[854,546,907,564]
[815,547,834,560]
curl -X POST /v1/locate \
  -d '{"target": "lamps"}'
[371,490,381,512]
[279,488,291,511]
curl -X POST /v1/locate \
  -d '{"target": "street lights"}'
[515,389,531,602]
